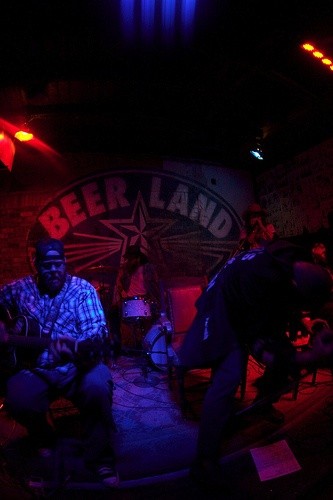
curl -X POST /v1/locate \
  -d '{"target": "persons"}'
[0,238,117,449]
[189,208,300,482]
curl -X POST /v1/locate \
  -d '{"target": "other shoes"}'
[95,464,119,488]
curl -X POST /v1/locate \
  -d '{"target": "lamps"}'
[249,141,265,161]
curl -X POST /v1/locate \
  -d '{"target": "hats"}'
[33,238,65,260]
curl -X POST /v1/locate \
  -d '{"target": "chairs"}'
[157,276,210,420]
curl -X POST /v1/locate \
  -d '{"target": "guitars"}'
[0,306,124,368]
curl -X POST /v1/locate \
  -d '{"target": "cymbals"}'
[86,266,116,273]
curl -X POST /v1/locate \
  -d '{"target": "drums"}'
[122,295,152,320]
[143,324,171,369]
